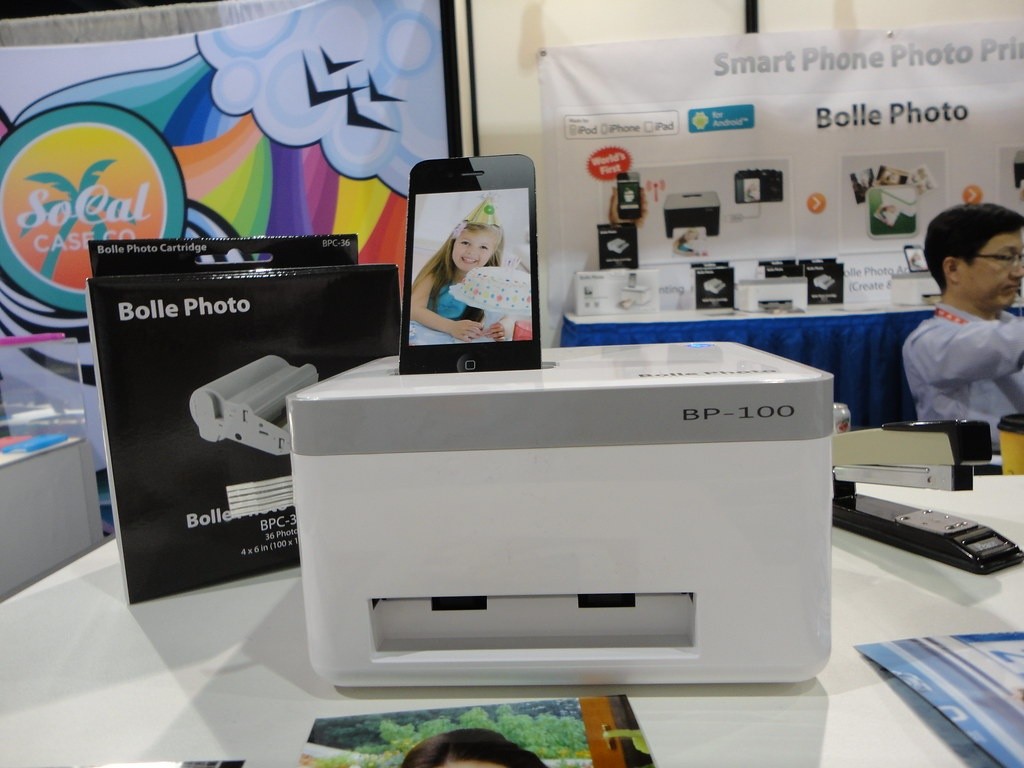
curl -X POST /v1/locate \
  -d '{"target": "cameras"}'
[665,192,721,238]
[734,169,783,205]
[285,342,835,688]
[892,273,942,306]
[735,276,807,313]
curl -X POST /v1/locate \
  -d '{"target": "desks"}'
[0,468,1024,767]
[562,307,1023,433]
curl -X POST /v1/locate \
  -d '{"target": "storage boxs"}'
[287,337,835,692]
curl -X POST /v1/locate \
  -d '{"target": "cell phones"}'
[398,153,542,375]
[904,246,929,274]
[617,172,641,219]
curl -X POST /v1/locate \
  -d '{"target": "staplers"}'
[832,419,1024,575]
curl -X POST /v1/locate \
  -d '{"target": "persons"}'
[912,169,933,194]
[909,254,927,270]
[400,729,547,768]
[410,218,513,344]
[876,205,897,225]
[900,204,1024,452]
[673,230,708,258]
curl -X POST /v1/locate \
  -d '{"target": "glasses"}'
[959,251,1024,268]
